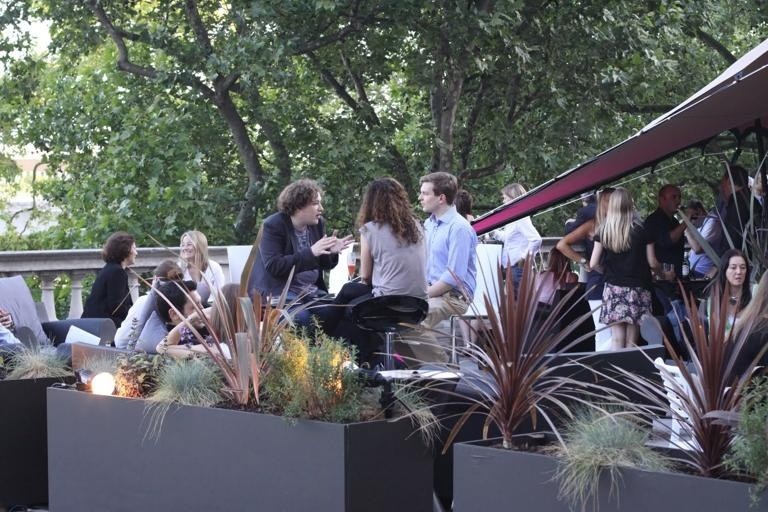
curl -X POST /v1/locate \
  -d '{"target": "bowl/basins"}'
[266,295,282,305]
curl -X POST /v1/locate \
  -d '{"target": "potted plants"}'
[0,327,79,512]
[373,159,767,512]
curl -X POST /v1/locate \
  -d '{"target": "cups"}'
[346,251,355,281]
[663,262,674,272]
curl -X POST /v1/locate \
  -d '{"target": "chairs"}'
[37,242,718,512]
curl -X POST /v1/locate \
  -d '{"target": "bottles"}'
[681,262,690,280]
[677,204,698,221]
[578,261,589,283]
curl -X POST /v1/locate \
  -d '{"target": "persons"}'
[490,185,543,289]
[84,231,241,359]
[246,171,478,367]
[537,162,768,352]
